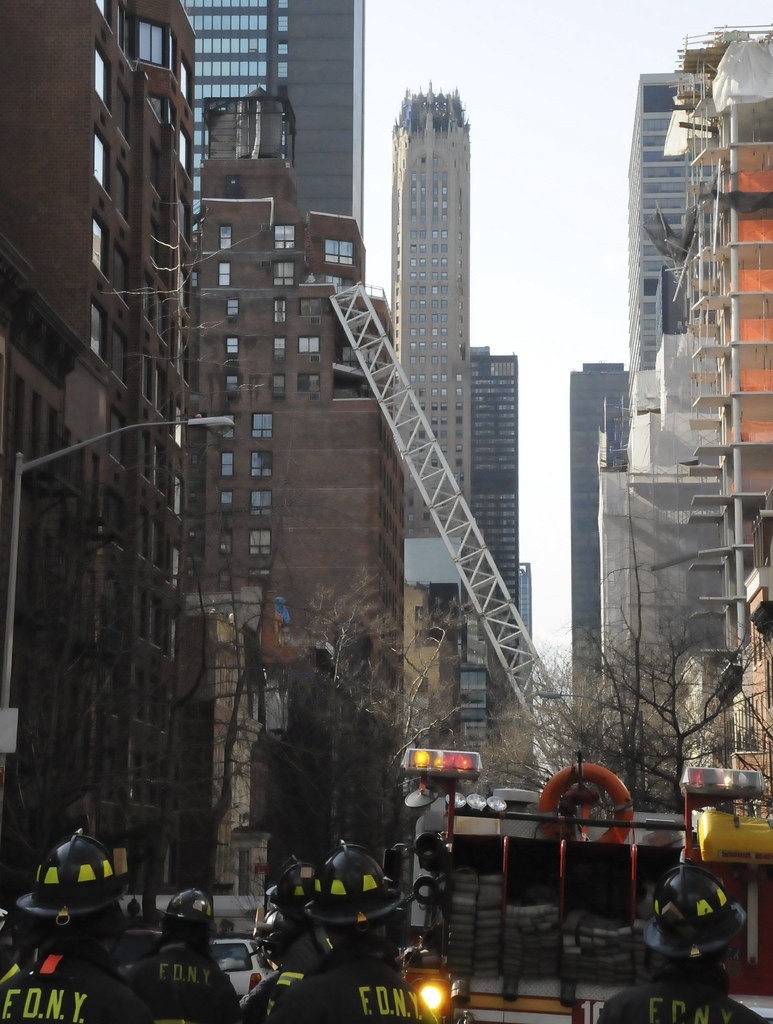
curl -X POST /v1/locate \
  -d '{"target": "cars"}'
[209,937,273,1001]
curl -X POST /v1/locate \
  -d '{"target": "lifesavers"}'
[536,764,633,844]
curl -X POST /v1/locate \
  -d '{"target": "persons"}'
[596,866,770,1024]
[0,822,436,1024]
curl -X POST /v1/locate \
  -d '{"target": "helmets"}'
[155,888,217,934]
[16,827,130,919]
[644,864,746,958]
[270,859,321,917]
[304,839,405,923]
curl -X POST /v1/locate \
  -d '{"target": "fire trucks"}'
[324,273,773,1023]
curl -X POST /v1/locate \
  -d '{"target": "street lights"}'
[0,416,237,805]
